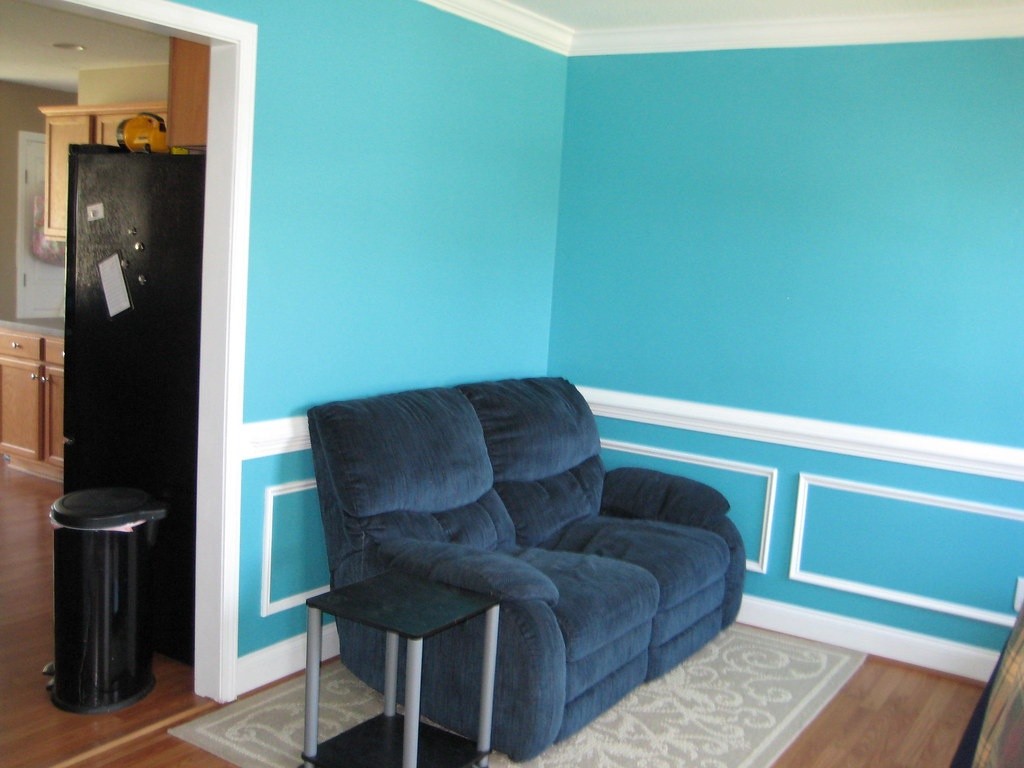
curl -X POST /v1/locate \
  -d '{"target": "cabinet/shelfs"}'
[40,101,169,241]
[0,327,64,483]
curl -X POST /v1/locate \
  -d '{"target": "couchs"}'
[306,377,746,765]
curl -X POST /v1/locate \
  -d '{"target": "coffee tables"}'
[306,568,499,768]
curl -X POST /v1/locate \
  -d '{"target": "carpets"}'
[166,621,866,768]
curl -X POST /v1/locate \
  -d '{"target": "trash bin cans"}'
[43,485,174,714]
